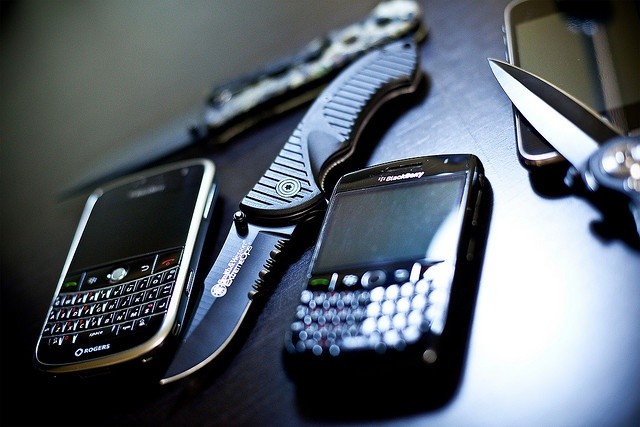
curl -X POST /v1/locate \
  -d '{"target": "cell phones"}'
[281,154,484,390]
[501,0,640,170]
[33,157,220,383]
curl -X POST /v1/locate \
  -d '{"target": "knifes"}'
[485,56,640,232]
[52,0,432,204]
[159,37,423,384]
